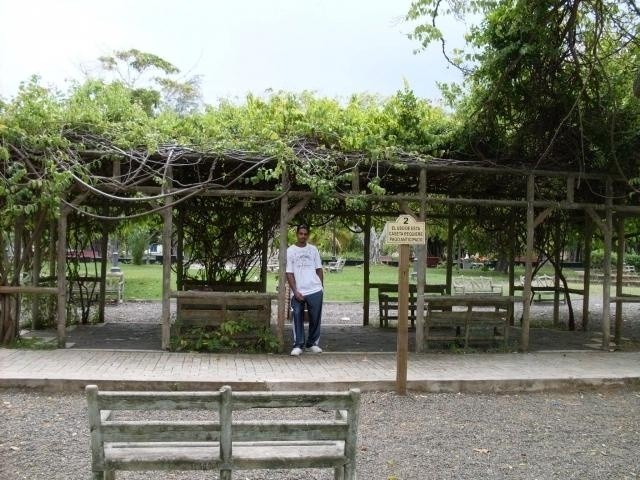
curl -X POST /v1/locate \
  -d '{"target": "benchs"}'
[369,276,514,350]
[520,274,567,304]
[66,272,124,304]
[610,265,640,286]
[85,383,361,480]
[168,281,277,348]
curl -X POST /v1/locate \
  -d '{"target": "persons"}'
[285,223,324,356]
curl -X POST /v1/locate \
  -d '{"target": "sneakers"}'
[305,346,322,353]
[290,348,303,356]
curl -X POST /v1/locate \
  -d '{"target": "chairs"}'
[266,255,280,273]
[324,258,347,273]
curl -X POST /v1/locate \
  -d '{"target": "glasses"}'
[298,224,309,228]
